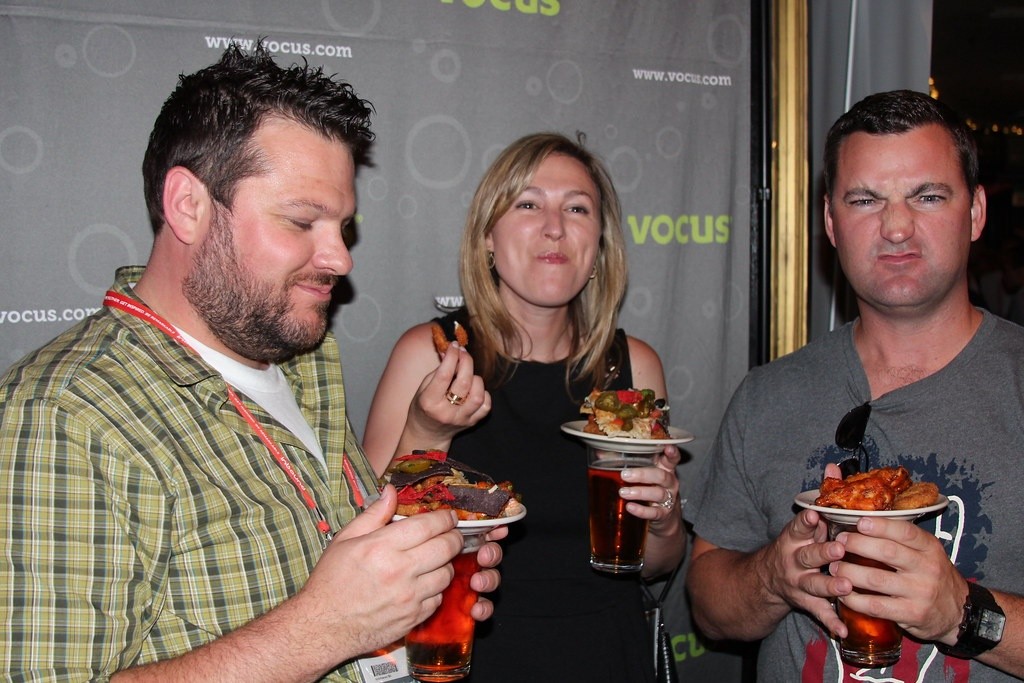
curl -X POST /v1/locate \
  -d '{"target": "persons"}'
[0,39,519,683]
[361,133,686,683]
[682,90,1023,683]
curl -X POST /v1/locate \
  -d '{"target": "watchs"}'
[932,580,1006,660]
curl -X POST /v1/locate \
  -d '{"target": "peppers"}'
[595,387,655,432]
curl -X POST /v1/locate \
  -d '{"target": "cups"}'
[827,520,917,669]
[584,443,656,573]
[403,533,485,683]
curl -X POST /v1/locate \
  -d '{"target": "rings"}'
[444,390,466,406]
[660,491,674,510]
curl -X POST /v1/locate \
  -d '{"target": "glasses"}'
[831,400,873,479]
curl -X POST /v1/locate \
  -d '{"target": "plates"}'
[794,489,950,526]
[560,419,697,454]
[362,494,528,535]
